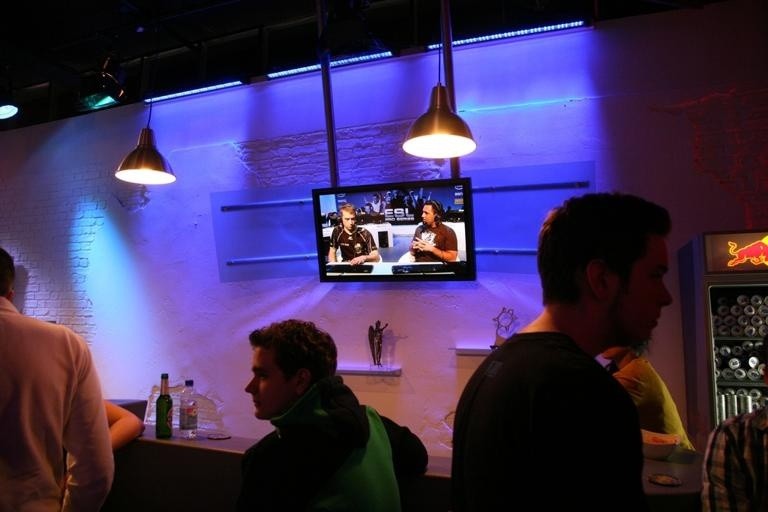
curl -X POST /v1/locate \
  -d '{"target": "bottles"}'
[179,379,198,440]
[155,373,173,439]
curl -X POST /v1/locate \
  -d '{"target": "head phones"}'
[430,200,444,221]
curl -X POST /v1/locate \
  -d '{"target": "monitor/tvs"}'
[310,176,478,283]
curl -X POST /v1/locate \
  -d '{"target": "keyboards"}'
[326,263,374,273]
[391,265,447,274]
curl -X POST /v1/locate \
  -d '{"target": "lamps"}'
[402,31,477,160]
[115,98,176,186]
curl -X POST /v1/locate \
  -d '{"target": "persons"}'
[446,188,674,512]
[699,334,768,510]
[409,200,458,262]
[101,400,145,452]
[355,189,452,224]
[328,204,380,267]
[602,332,693,449]
[240,318,429,511]
[0,246,114,510]
